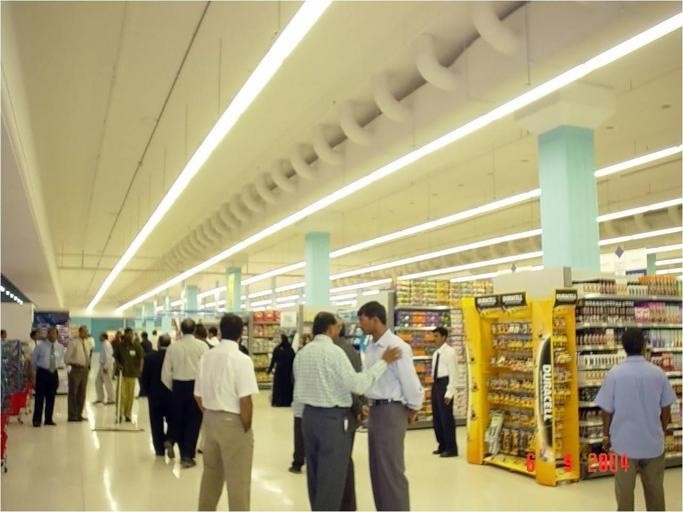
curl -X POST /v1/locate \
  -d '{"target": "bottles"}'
[572,274,682,465]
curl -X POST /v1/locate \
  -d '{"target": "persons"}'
[338,322,368,511]
[592,327,677,511]
[430,326,459,457]
[288,401,306,472]
[357,301,425,511]
[292,311,403,511]
[267,334,295,407]
[192,311,259,511]
[1,318,219,467]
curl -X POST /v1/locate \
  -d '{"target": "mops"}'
[90,369,144,433]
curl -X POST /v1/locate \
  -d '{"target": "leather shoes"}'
[433,449,457,457]
[33,420,56,427]
[69,416,87,421]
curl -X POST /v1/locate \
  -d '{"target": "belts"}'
[373,400,400,404]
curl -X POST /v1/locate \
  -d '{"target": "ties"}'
[50,345,56,372]
[82,339,89,369]
[434,353,440,381]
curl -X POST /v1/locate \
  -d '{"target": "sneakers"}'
[181,457,196,466]
[164,440,174,458]
[289,467,300,473]
